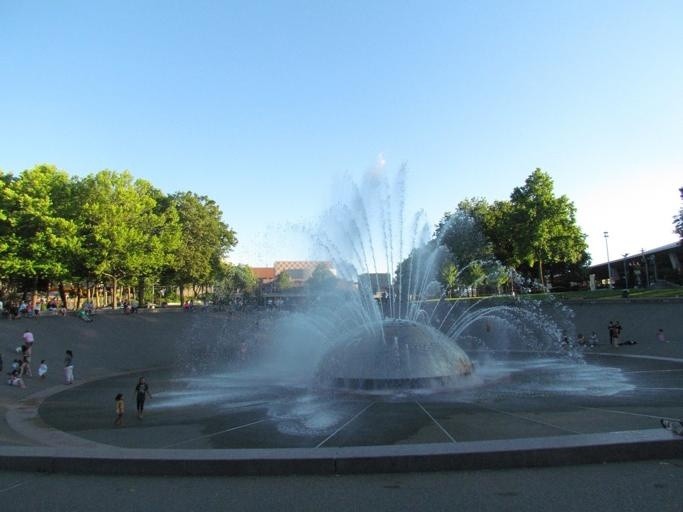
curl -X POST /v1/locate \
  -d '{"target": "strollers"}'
[78,309,94,322]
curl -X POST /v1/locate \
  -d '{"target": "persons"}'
[183,299,193,312]
[560,330,600,349]
[84,300,96,315]
[123,298,139,315]
[115,393,126,427]
[8,330,74,388]
[135,376,152,420]
[655,328,667,344]
[608,319,637,346]
[3,296,67,320]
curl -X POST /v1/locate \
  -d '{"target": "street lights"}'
[622,252,629,290]
[603,230,612,291]
